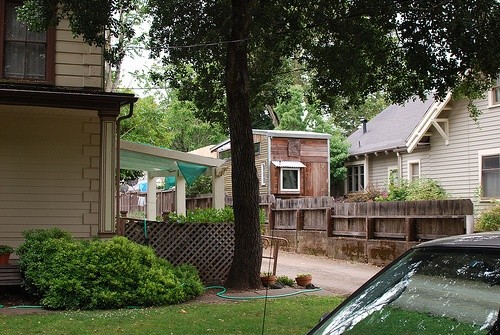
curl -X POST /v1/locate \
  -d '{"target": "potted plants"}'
[260,271,275,287]
[0,245,15,264]
[295,273,312,287]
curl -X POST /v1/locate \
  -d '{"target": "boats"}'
[306,230,500,335]
